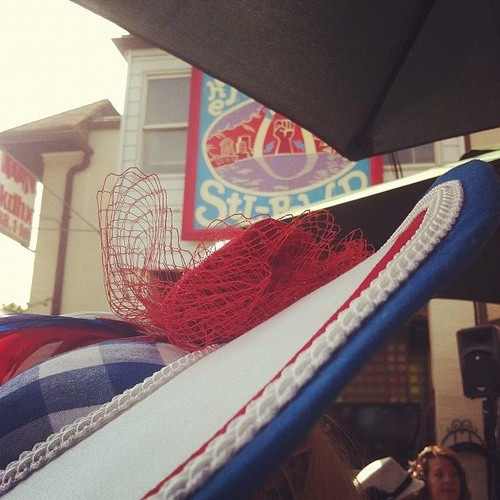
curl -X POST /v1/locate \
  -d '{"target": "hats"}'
[1,159,496,500]
[354,456,424,500]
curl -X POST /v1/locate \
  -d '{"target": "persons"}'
[0,155,496,500]
[412,445,472,500]
[353,456,425,500]
